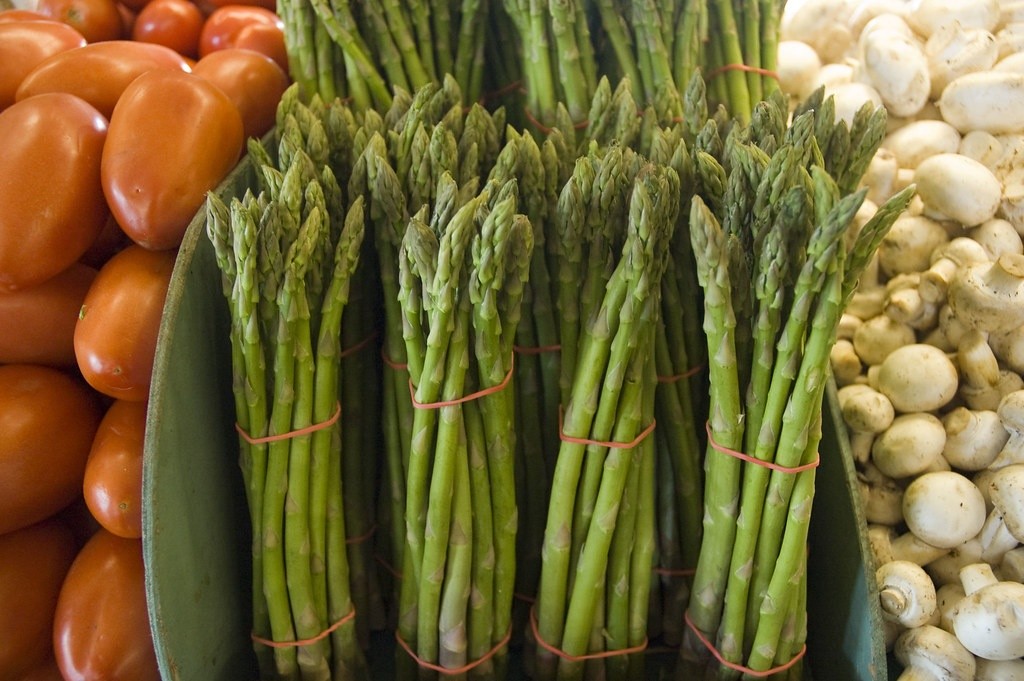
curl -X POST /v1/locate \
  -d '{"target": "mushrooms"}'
[772,0,1024,681]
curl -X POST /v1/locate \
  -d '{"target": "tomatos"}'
[0,0,289,681]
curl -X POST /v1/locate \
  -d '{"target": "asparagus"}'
[203,0,919,681]
